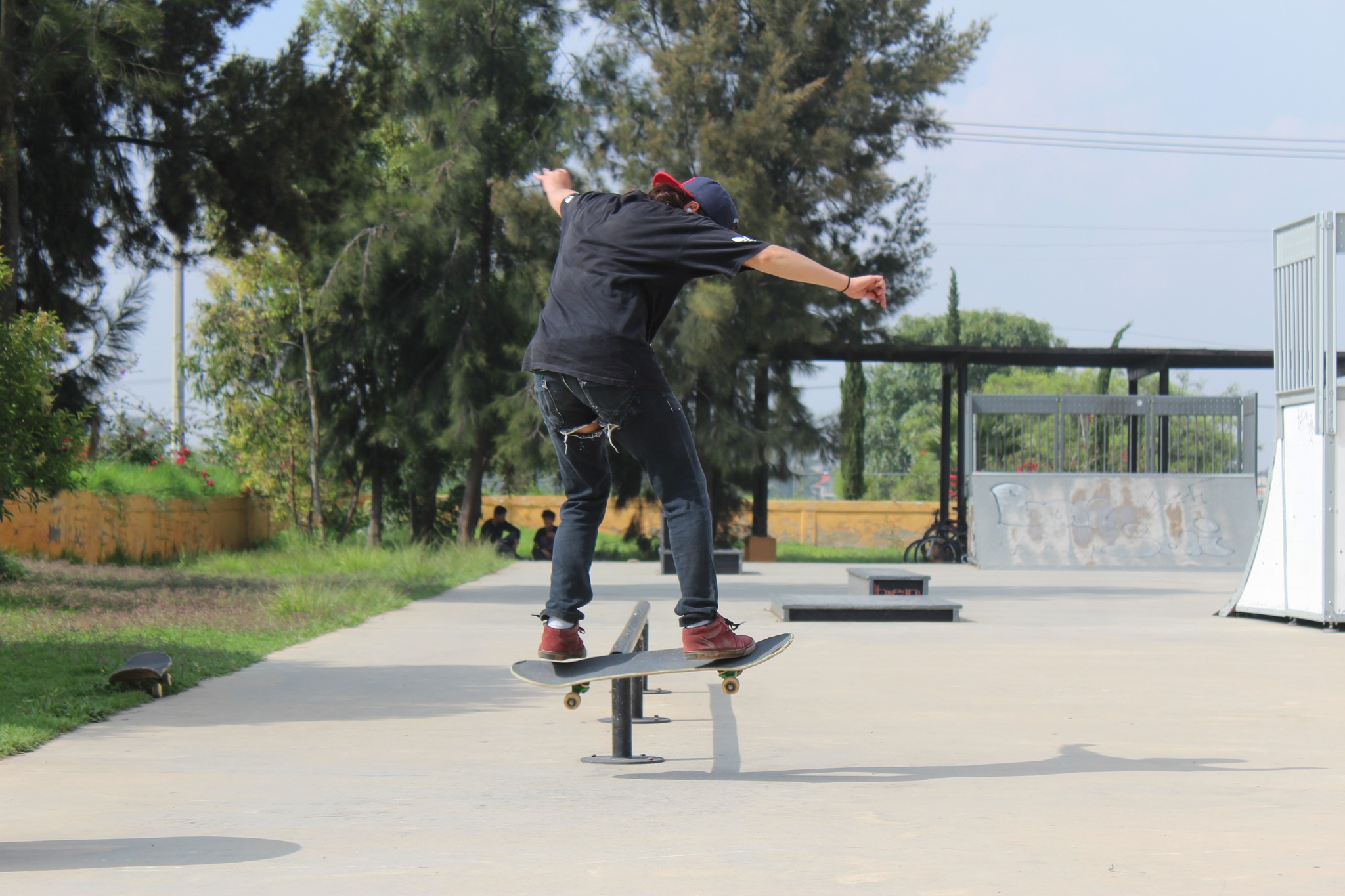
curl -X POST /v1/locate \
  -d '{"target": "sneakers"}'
[538,623,587,661]
[682,612,756,660]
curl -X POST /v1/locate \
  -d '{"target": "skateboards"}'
[510,632,795,709]
[108,650,173,698]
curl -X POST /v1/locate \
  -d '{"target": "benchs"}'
[770,594,963,623]
[847,567,930,595]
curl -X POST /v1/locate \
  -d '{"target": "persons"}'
[532,510,558,560]
[480,505,524,560]
[516,168,889,658]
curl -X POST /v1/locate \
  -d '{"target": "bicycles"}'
[903,505,968,566]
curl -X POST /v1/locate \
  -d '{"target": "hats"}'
[653,172,739,231]
